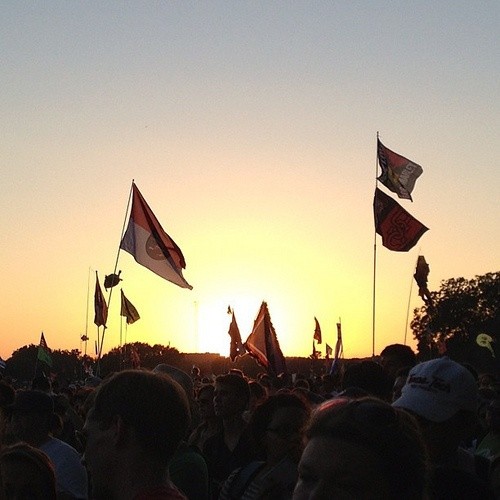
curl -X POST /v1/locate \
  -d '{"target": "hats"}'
[151,365,194,400]
[4,390,54,414]
[390,356,478,424]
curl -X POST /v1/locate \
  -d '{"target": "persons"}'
[0,344,500,500]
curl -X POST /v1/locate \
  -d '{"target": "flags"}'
[94,279,108,329]
[229,316,245,362]
[314,318,321,344]
[120,291,140,324]
[38,335,49,363]
[377,139,422,202]
[374,188,430,252]
[244,303,286,374]
[124,184,193,290]
